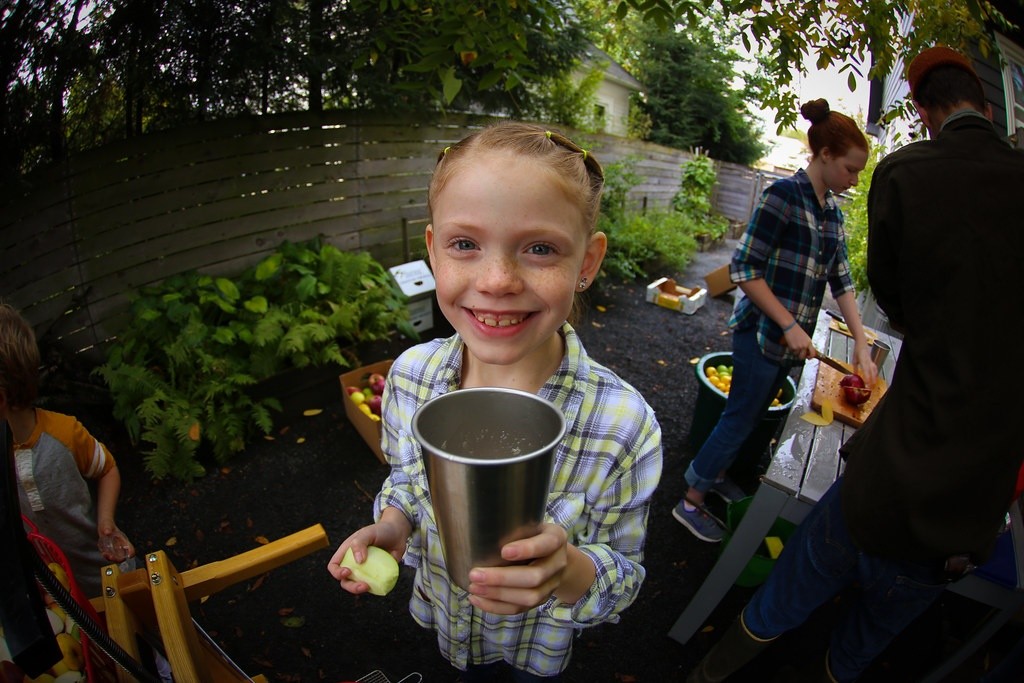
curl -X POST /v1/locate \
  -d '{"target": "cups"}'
[871,340,890,376]
[98,531,128,558]
[411,387,569,592]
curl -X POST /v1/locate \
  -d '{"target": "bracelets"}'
[783,320,796,332]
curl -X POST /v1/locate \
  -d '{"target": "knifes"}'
[826,311,846,324]
[808,348,853,376]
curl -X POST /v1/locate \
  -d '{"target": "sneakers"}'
[672,491,728,542]
[709,476,746,505]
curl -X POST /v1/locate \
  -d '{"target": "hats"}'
[908,47,979,97]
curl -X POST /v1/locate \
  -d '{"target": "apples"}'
[843,386,871,404]
[346,373,386,422]
[339,546,399,596]
[840,373,865,388]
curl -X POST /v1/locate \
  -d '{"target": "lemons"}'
[838,322,848,330]
[705,365,783,407]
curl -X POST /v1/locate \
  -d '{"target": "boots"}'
[773,644,838,683]
[686,605,784,683]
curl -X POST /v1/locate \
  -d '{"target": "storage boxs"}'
[340,358,395,465]
[646,277,709,315]
[387,259,436,340]
[705,264,738,299]
[726,217,748,240]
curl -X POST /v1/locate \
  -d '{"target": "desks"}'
[664,309,1024,683]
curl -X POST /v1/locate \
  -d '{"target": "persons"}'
[0,303,172,683]
[671,46,1024,683]
[327,122,663,683]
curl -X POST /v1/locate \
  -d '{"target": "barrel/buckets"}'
[689,351,796,463]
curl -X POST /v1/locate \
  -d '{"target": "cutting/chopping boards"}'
[811,354,887,428]
[829,317,878,346]
